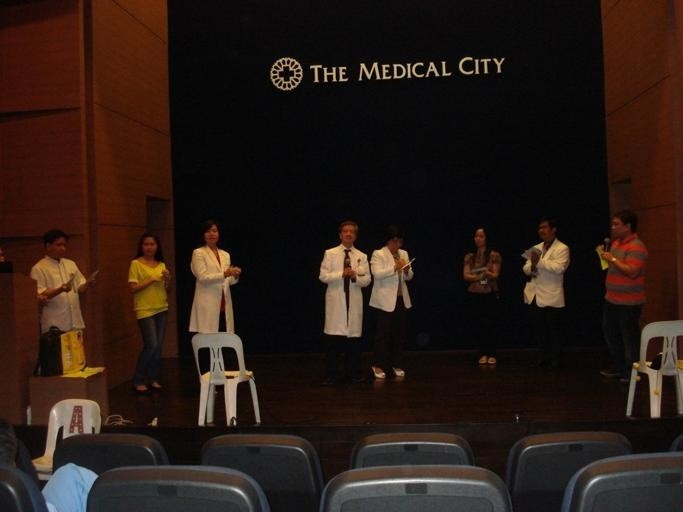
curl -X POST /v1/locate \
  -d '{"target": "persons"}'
[125,228,172,394]
[28,227,101,376]
[186,217,242,338]
[521,216,570,373]
[459,226,503,365]
[596,209,649,384]
[368,224,414,379]
[316,219,372,384]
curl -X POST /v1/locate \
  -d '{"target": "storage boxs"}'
[28,373,108,425]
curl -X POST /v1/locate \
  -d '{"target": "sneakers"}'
[479,356,488,364]
[488,357,496,364]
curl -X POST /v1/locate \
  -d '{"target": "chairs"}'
[349,430,476,468]
[563,451,682,512]
[623,318,683,418]
[190,331,262,429]
[198,432,322,511]
[85,464,271,512]
[320,465,511,511]
[506,432,632,511]
[29,399,105,481]
[0,423,166,511]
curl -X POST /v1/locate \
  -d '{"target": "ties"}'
[344,249,350,290]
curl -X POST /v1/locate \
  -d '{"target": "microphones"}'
[346,259,351,288]
[604,237,610,252]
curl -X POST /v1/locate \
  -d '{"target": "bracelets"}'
[610,257,616,264]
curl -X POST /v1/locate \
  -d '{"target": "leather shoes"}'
[148,382,165,392]
[131,382,152,396]
[392,366,405,377]
[372,367,386,379]
